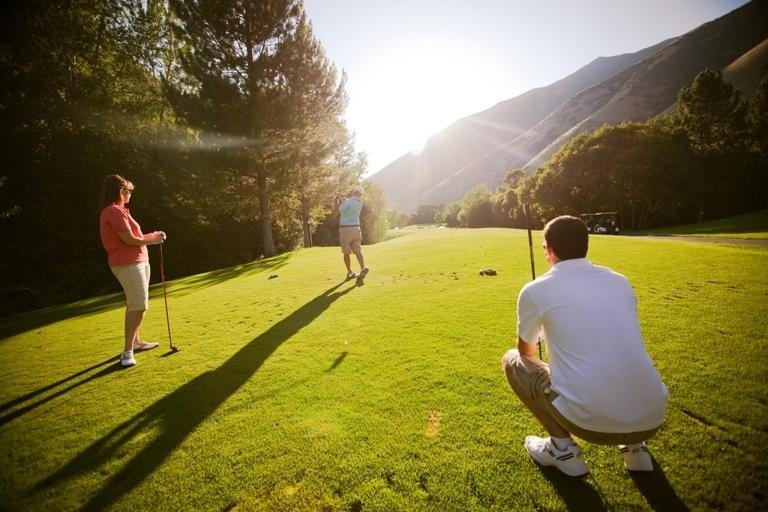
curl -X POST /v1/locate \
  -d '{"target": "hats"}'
[353,187,364,195]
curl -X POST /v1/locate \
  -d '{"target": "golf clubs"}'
[160,242,180,351]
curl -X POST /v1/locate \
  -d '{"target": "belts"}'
[340,224,360,228]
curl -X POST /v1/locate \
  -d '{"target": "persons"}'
[502,213,671,477]
[98,174,169,368]
[332,185,369,285]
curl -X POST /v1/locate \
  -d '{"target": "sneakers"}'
[119,350,136,367]
[619,441,654,473]
[524,433,589,478]
[356,268,369,280]
[347,272,358,279]
[133,341,159,352]
